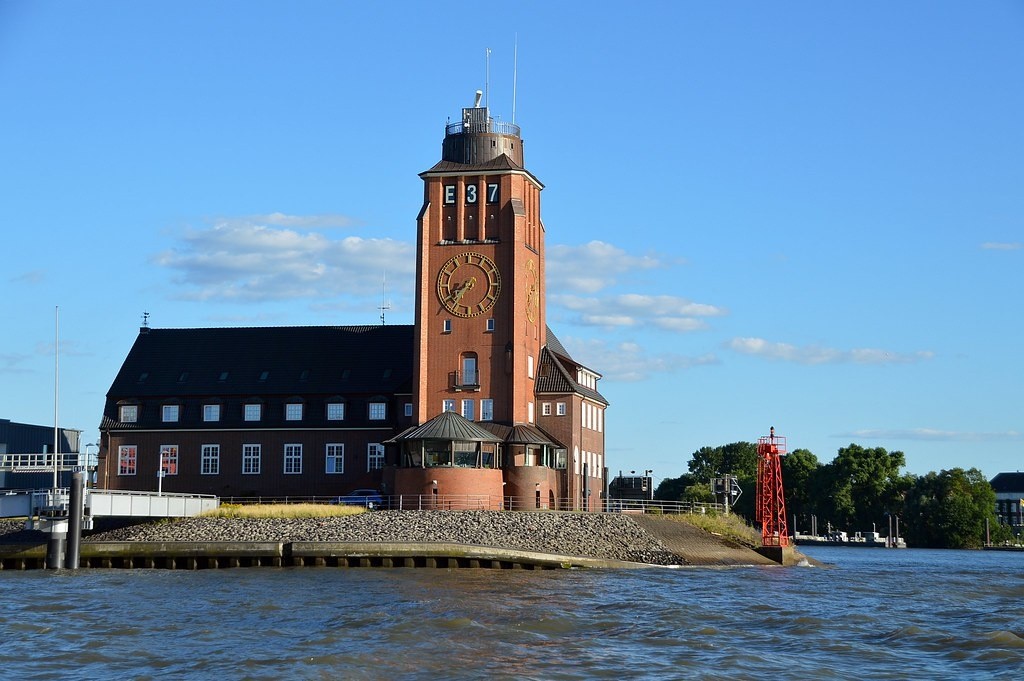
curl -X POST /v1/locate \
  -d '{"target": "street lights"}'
[84,443,95,489]
[158,450,167,496]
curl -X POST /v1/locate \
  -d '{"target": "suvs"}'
[330,489,386,510]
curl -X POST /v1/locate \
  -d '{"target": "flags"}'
[1019,498,1024,508]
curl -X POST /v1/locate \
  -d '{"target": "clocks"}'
[437,251,502,319]
